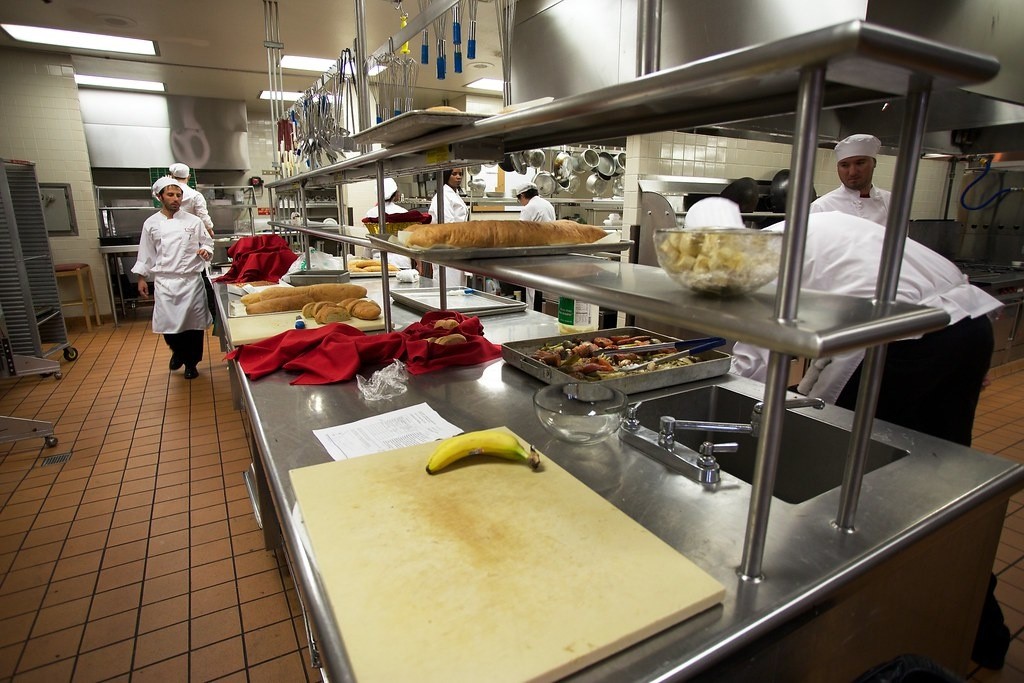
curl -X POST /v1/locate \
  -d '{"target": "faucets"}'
[657,397,826,447]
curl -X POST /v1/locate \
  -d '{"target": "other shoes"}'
[972,626,1010,670]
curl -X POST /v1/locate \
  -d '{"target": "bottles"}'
[401,193,404,201]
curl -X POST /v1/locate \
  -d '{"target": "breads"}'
[348,260,399,272]
[402,220,608,248]
[240,284,380,325]
[424,319,467,345]
[425,106,460,112]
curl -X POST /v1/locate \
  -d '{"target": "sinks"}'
[604,384,912,506]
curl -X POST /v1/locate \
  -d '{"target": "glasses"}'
[517,196,521,203]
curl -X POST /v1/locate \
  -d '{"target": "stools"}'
[55,264,101,332]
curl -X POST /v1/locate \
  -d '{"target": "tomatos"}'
[536,337,672,372]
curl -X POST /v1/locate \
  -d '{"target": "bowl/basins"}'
[533,382,627,443]
[653,228,784,296]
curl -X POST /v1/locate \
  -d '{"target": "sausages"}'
[617,336,651,345]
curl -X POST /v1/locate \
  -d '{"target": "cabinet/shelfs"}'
[94,184,258,209]
[264,21,1000,360]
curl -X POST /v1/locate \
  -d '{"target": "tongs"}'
[276,47,358,175]
[590,337,726,372]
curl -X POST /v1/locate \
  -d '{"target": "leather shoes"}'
[184,366,198,379]
[170,352,183,370]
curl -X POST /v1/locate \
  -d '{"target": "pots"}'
[719,168,818,224]
[523,145,626,196]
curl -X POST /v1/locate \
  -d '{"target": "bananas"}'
[425,429,540,473]
[659,228,748,284]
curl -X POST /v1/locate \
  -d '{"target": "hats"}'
[683,198,747,230]
[834,134,881,165]
[384,177,398,201]
[169,163,189,178]
[152,177,183,201]
[516,182,537,196]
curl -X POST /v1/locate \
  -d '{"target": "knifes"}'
[295,315,305,329]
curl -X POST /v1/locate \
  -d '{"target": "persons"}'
[807,134,909,238]
[364,178,417,273]
[516,182,556,313]
[428,168,469,286]
[131,177,215,380]
[683,197,1005,449]
[169,163,214,290]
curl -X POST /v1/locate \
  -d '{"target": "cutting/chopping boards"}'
[288,426,724,683]
[228,299,395,346]
